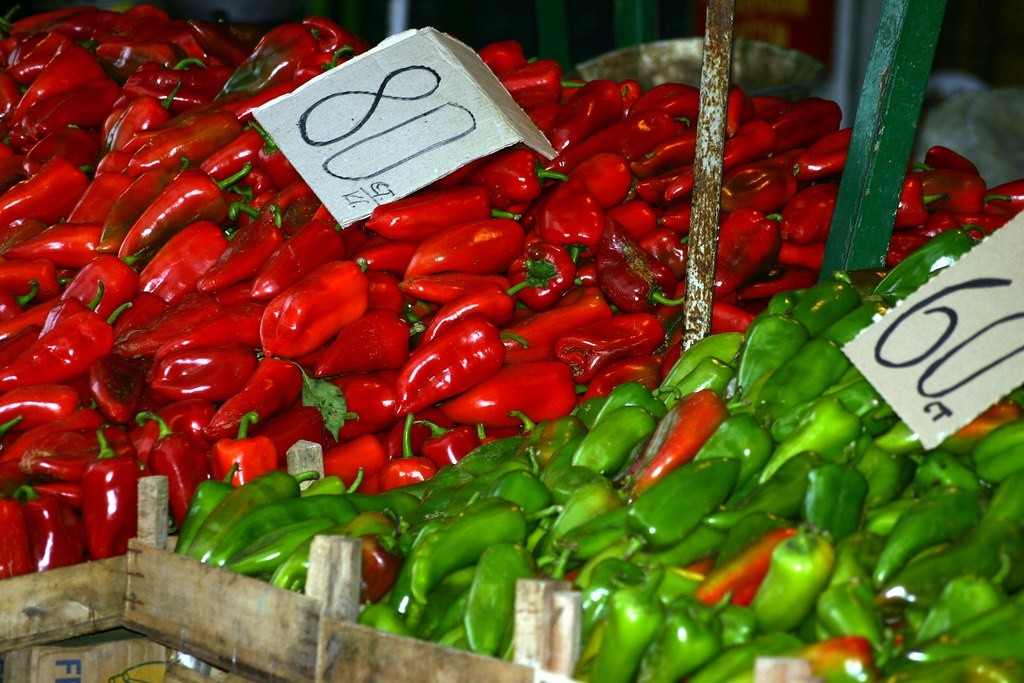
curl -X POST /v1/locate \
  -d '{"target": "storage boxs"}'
[0,479,584,683]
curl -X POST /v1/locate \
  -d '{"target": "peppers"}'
[164,219,1024,683]
[0,3,1024,582]
